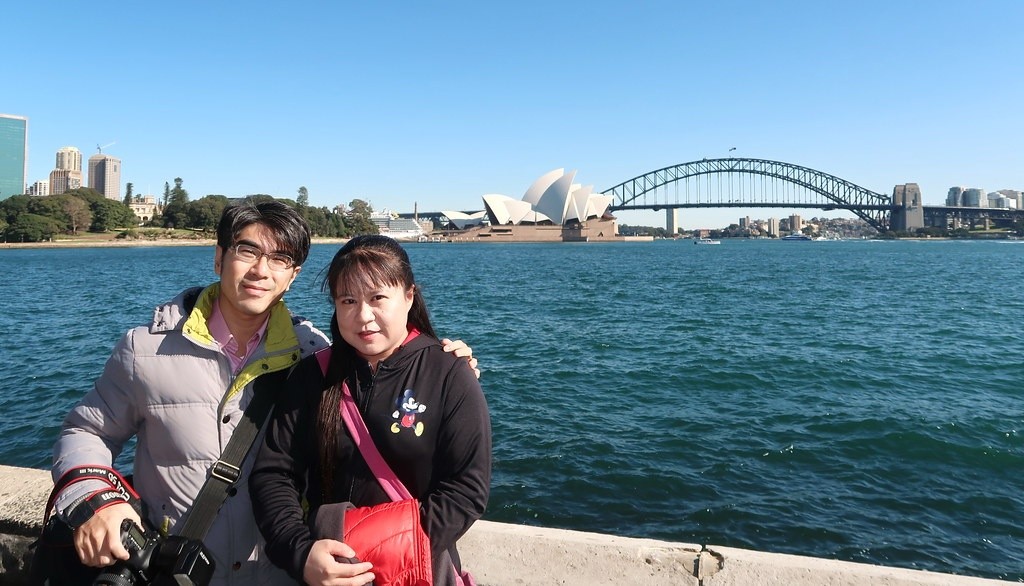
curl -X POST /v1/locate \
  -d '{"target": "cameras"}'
[94,518,216,586]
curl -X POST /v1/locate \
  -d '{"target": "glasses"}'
[224,242,297,271]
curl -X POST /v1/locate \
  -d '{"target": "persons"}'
[52,195,480,586]
[247,235,493,586]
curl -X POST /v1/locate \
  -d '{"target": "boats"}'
[693,237,721,245]
[815,236,841,241]
[780,233,812,241]
